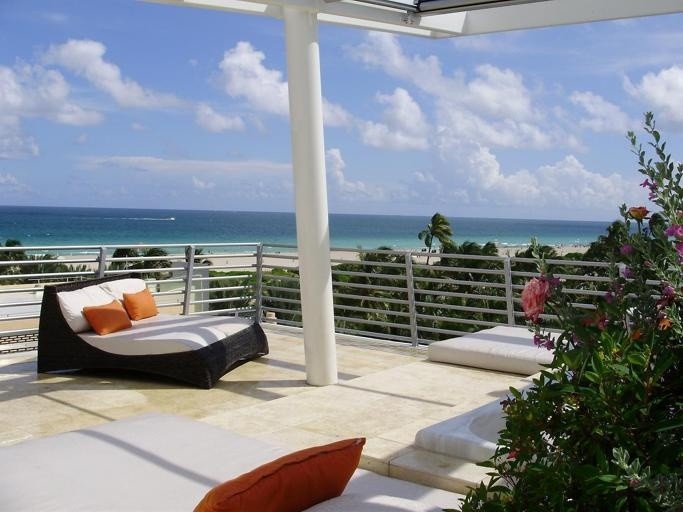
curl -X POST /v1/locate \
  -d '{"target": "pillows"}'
[54,285,114,333]
[83,299,133,336]
[122,289,158,320]
[100,279,147,300]
[192,436,365,510]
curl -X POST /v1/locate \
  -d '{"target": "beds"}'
[0,408,475,511]
[37,273,270,390]
